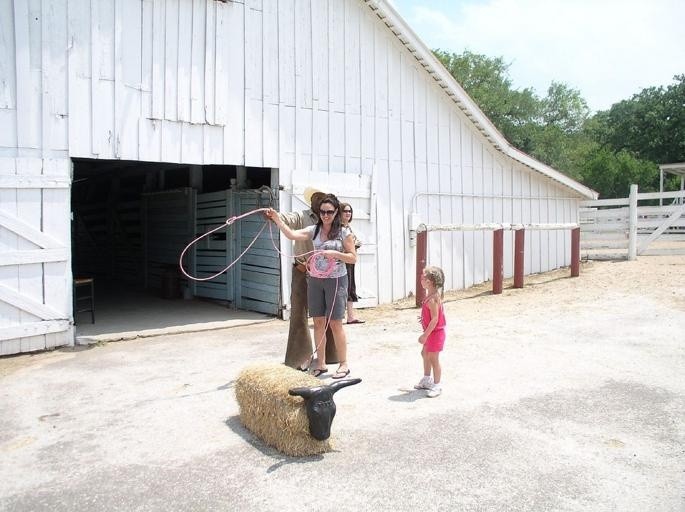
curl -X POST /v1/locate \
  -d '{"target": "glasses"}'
[342,209,352,213]
[319,208,336,215]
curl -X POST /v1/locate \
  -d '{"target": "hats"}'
[304,187,328,206]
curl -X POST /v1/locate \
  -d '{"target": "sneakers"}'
[414,375,442,397]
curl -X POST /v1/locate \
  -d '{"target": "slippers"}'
[332,369,351,379]
[312,367,328,377]
[346,320,367,325]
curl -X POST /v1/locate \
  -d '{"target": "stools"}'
[73,275,96,326]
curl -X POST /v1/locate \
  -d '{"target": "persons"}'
[339,203,367,324]
[266,194,357,378]
[414,266,448,397]
[263,187,341,371]
